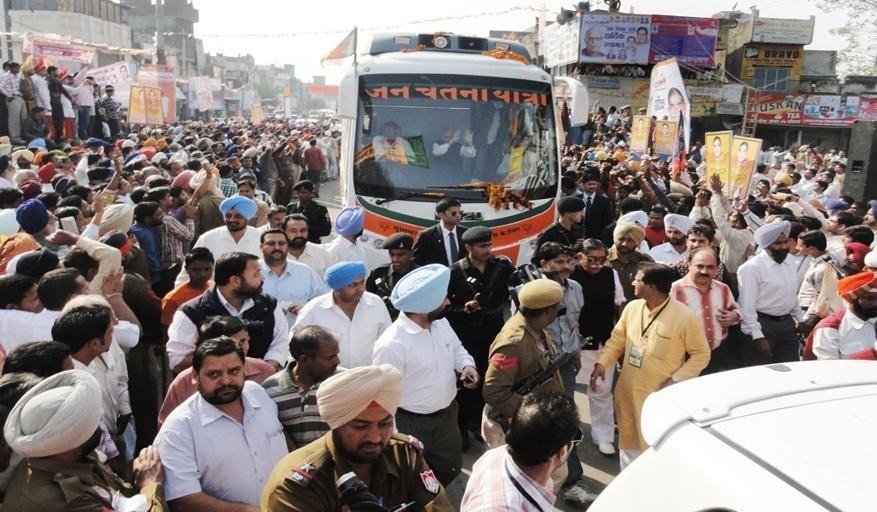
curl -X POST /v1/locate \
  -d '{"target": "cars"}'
[243,107,334,129]
[578,356,877,512]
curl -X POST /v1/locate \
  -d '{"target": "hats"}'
[218,193,258,221]
[98,229,129,249]
[516,278,566,311]
[323,261,369,292]
[16,249,60,285]
[1,369,104,459]
[334,205,366,238]
[0,118,343,196]
[834,270,877,304]
[20,54,68,83]
[314,362,406,432]
[461,225,494,244]
[15,198,50,235]
[388,262,453,316]
[556,104,877,250]
[379,231,413,251]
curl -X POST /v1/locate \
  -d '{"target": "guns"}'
[489,336,594,420]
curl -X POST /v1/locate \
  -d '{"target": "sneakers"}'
[563,484,599,505]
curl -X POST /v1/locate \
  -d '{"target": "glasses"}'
[569,425,586,447]
[445,210,464,216]
[582,254,608,264]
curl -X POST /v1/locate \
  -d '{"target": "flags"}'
[320,29,356,69]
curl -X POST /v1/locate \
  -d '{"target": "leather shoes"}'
[592,437,616,455]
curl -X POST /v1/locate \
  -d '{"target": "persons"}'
[617,48,627,60]
[579,25,603,58]
[606,47,616,59]
[635,26,649,48]
[626,36,635,51]
[0,51,877,511]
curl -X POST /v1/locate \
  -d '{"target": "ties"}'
[449,232,461,264]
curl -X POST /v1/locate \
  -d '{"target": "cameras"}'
[334,470,424,512]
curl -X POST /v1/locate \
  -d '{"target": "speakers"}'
[843,119,876,201]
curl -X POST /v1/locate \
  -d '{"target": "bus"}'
[335,22,590,280]
